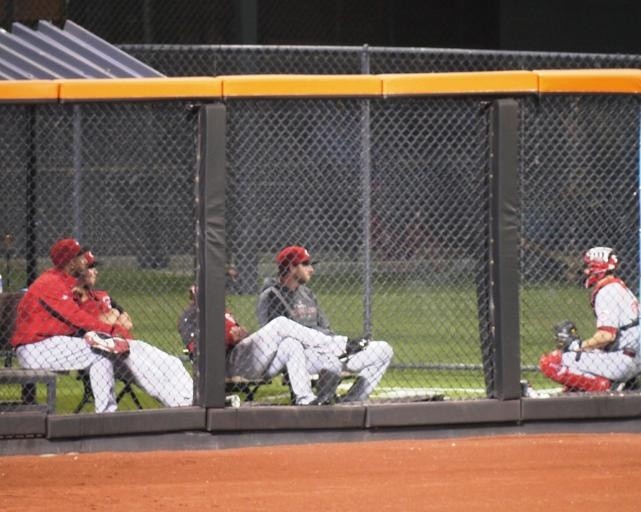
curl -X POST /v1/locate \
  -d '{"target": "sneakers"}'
[611,377,637,392]
[309,395,329,405]
[225,395,240,408]
[338,334,369,360]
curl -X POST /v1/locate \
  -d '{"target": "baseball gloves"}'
[553,317,582,354]
[83,330,130,356]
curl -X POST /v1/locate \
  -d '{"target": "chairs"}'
[1,292,143,410]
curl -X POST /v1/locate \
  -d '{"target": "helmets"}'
[583,247,619,288]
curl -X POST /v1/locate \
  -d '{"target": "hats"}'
[50,239,104,271]
[279,246,320,266]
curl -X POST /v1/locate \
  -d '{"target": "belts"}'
[624,350,635,357]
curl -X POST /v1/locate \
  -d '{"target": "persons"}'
[176,283,368,406]
[11,240,121,414]
[257,245,393,402]
[73,245,241,407]
[540,246,640,392]
